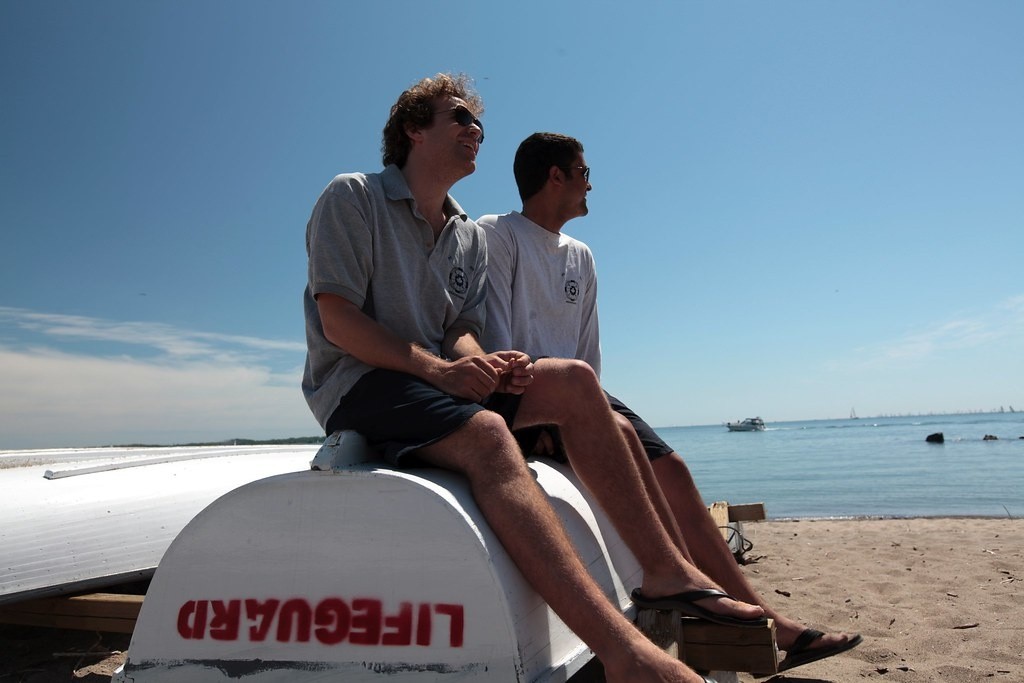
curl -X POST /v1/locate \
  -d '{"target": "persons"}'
[474,133,863,671]
[301,74,766,683]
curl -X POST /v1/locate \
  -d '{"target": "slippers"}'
[630,582,768,628]
[750,628,862,679]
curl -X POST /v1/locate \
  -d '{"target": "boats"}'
[113,461,646,683]
[0,443,321,607]
[726,416,766,432]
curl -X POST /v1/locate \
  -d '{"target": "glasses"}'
[560,166,590,182]
[432,105,485,144]
